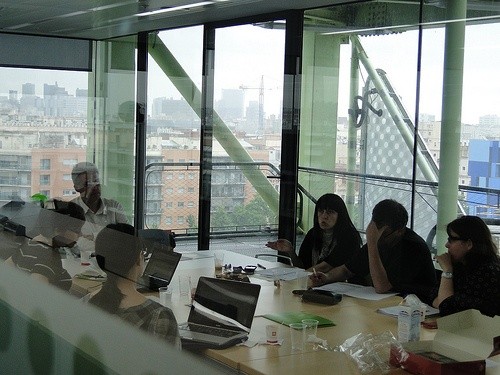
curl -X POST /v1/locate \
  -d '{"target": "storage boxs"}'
[389,308,500,375]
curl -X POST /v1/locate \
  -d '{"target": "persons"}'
[265,193,364,273]
[0,199,85,294]
[78,223,182,354]
[310,200,438,294]
[432,216,500,317]
[65,162,130,227]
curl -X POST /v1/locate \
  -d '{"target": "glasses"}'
[138,248,147,255]
[448,236,468,243]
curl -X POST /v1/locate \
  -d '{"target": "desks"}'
[70,249,500,375]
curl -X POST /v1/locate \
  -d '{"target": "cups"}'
[272,269,284,288]
[81,251,90,265]
[289,323,308,350]
[266,324,280,344]
[158,286,173,307]
[178,274,204,304]
[296,271,308,290]
[301,319,319,343]
[214,252,224,271]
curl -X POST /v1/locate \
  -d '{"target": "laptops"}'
[176,276,261,347]
[136,247,182,293]
[138,229,171,259]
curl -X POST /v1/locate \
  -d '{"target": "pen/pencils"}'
[257,264,266,270]
[313,268,319,280]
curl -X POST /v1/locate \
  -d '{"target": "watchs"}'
[442,272,453,278]
